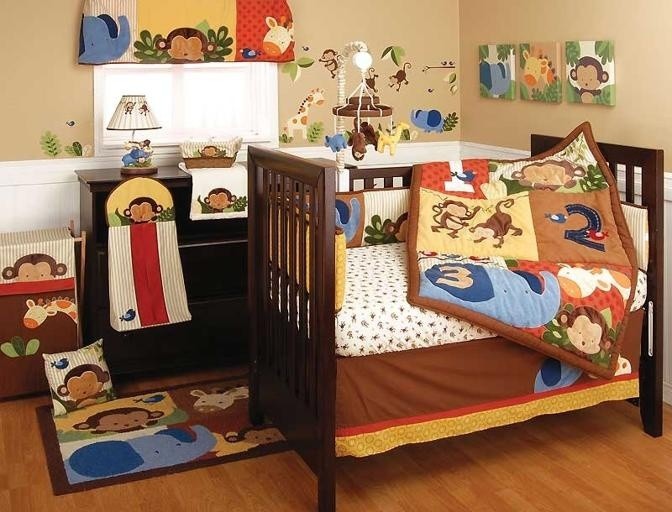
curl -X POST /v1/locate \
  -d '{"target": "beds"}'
[246,134,664,512]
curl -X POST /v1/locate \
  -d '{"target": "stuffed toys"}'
[323,116,411,162]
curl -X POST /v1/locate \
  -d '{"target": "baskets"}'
[184,151,238,169]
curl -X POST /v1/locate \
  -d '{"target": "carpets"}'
[34,374,297,496]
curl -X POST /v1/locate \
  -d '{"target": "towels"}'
[0,227,75,296]
[108,222,192,332]
[178,162,248,221]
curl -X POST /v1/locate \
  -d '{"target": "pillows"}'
[43,338,117,415]
[270,185,411,315]
[621,200,649,274]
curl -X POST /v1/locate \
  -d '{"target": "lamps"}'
[106,94,163,174]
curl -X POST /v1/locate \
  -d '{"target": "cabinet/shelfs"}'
[74,167,248,382]
